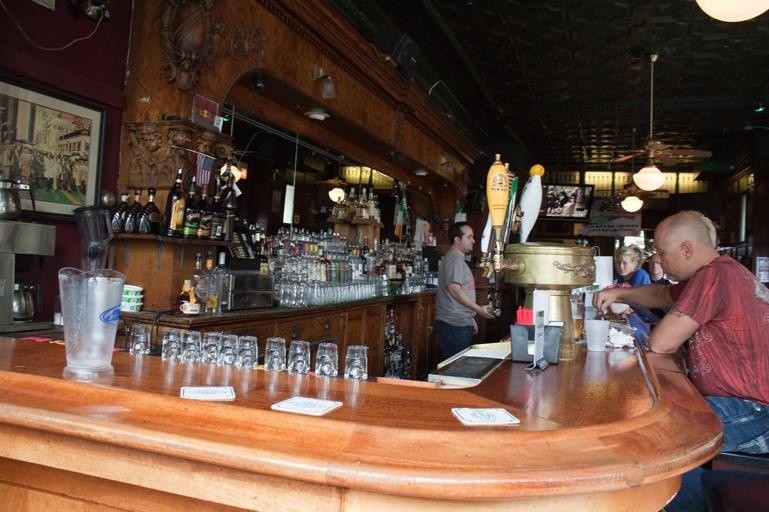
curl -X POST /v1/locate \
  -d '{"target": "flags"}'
[196,152,214,187]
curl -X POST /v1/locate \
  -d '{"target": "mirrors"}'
[224,113,438,247]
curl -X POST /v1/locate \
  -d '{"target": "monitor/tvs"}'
[537,183,595,222]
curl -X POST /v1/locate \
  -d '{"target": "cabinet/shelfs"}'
[275,306,344,379]
[386,292,438,383]
[229,317,275,363]
[121,315,229,366]
[343,301,384,381]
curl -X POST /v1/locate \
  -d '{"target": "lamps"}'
[631,156,667,194]
[695,0,768,25]
[621,190,648,214]
[325,184,352,204]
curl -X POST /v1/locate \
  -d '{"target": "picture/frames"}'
[0,68,111,225]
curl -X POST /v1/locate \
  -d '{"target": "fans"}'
[611,51,715,169]
[606,126,674,199]
[305,157,356,187]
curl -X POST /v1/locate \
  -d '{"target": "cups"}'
[424,233,432,246]
[127,322,368,381]
[277,279,378,309]
[56,267,127,381]
[571,302,586,342]
[584,319,609,352]
[379,275,427,296]
[580,351,607,385]
[277,257,310,284]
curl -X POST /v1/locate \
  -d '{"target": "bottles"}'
[176,249,235,317]
[110,169,225,241]
[383,308,412,379]
[242,219,364,280]
[363,234,430,278]
[333,187,381,224]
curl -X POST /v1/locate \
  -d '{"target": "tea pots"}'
[73,204,117,244]
[13,283,42,319]
[78,240,109,270]
[0,177,36,220]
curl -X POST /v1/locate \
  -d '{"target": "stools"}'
[706,443,767,480]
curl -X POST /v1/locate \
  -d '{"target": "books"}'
[427,355,504,387]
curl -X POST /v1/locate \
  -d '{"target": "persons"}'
[4,142,87,195]
[592,212,769,512]
[613,244,652,329]
[687,210,717,250]
[434,221,495,360]
[646,255,673,331]
